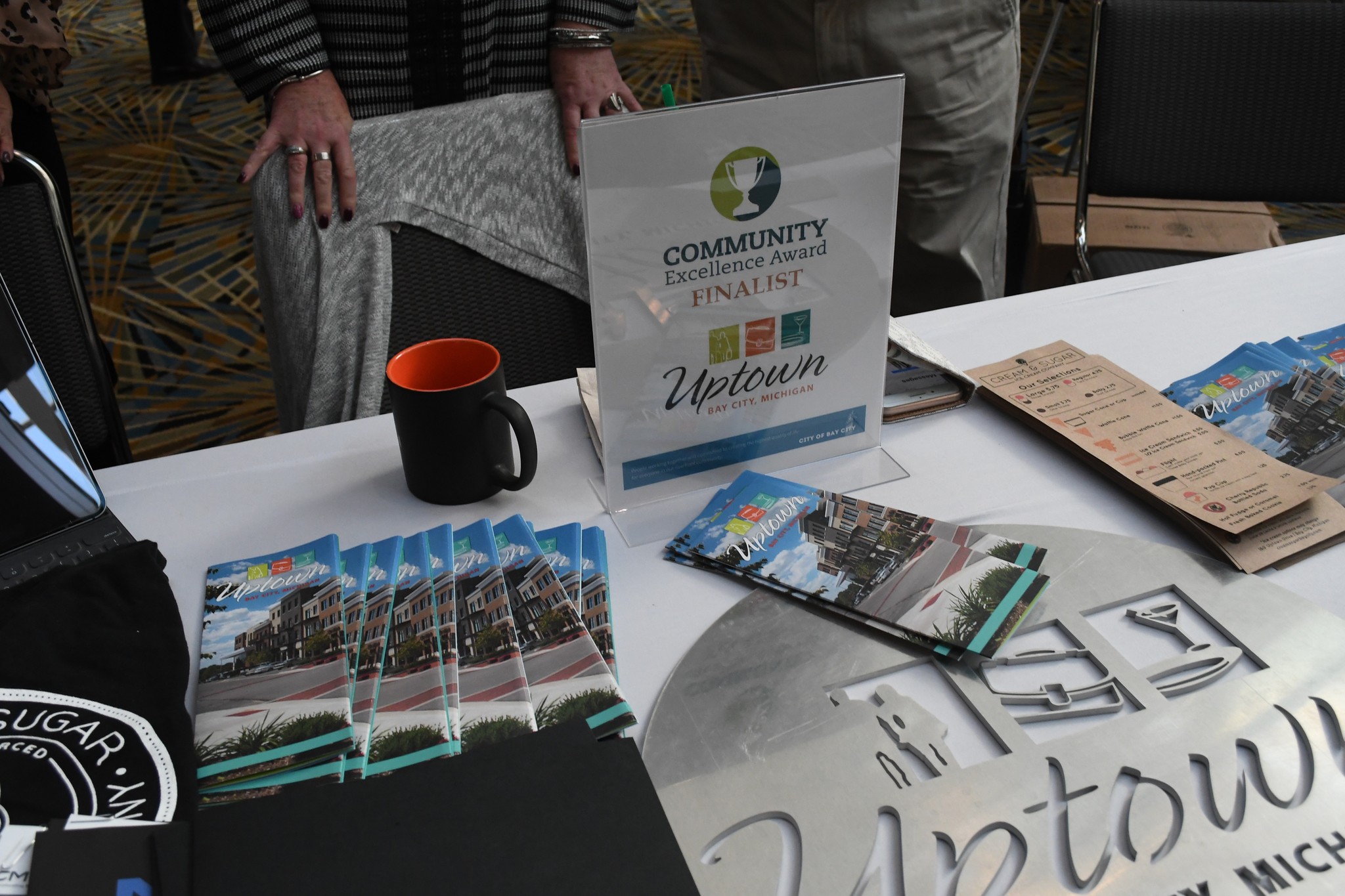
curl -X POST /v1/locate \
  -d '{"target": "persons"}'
[0,0,72,184]
[691,0,1024,306]
[197,0,647,432]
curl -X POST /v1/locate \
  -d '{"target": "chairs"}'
[259,90,597,430]
[1066,0,1345,282]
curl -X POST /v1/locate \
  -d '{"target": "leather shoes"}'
[152,56,225,84]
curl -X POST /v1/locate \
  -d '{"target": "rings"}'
[284,145,308,159]
[310,151,330,162]
[605,91,625,112]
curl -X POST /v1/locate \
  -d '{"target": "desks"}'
[0,234,1344,896]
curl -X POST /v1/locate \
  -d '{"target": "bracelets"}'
[546,24,613,49]
[265,68,326,103]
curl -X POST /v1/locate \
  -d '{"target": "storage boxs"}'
[1021,176,1285,293]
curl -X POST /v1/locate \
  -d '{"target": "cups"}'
[388,337,536,505]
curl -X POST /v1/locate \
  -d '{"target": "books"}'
[1159,324,1345,502]
[191,513,641,801]
[660,471,1051,670]
